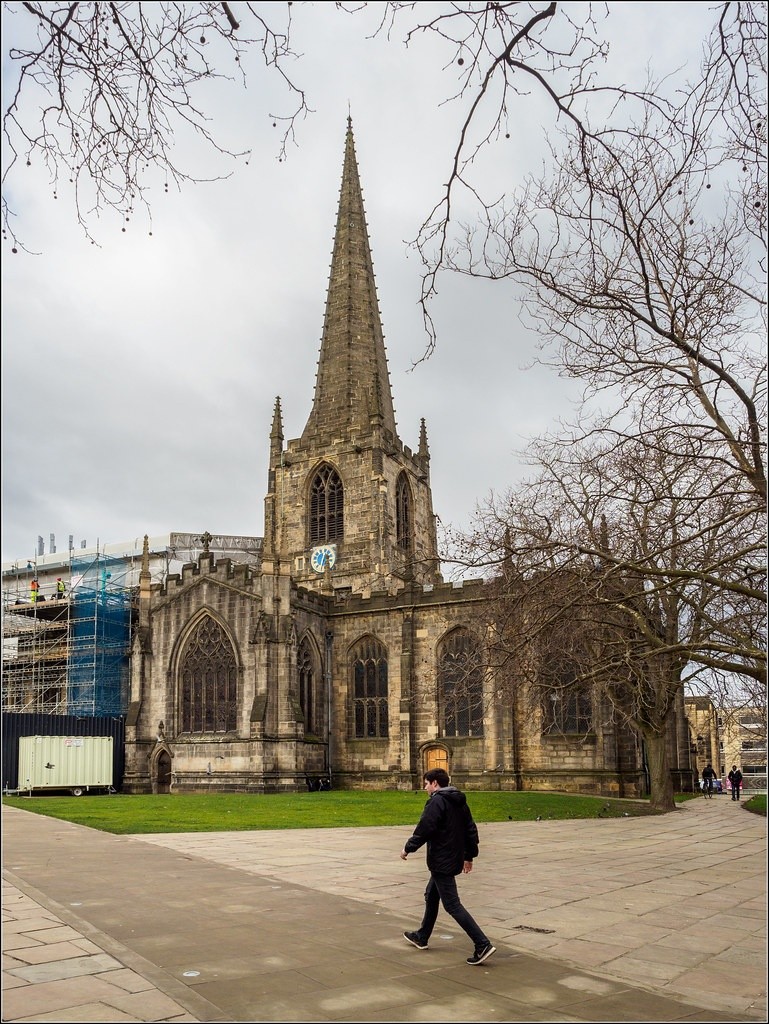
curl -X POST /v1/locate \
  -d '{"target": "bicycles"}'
[702,778,717,799]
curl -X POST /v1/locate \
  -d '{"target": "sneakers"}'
[466,942,496,965]
[402,931,429,950]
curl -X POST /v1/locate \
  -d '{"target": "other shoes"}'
[732,798,737,801]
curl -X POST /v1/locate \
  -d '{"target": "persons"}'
[702,764,717,795]
[30,577,40,602]
[400,767,497,965]
[57,578,66,599]
[728,765,743,801]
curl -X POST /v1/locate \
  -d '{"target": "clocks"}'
[311,547,336,572]
